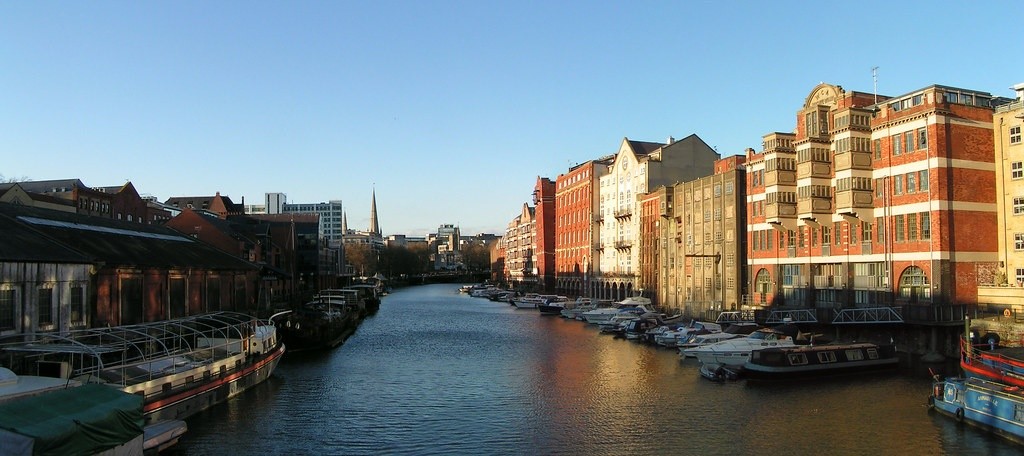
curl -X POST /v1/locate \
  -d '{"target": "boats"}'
[269,284,382,350]
[683,329,797,366]
[741,340,901,378]
[458,281,742,358]
[960,316,1024,389]
[0,310,287,430]
[701,363,738,383]
[927,376,1024,447]
